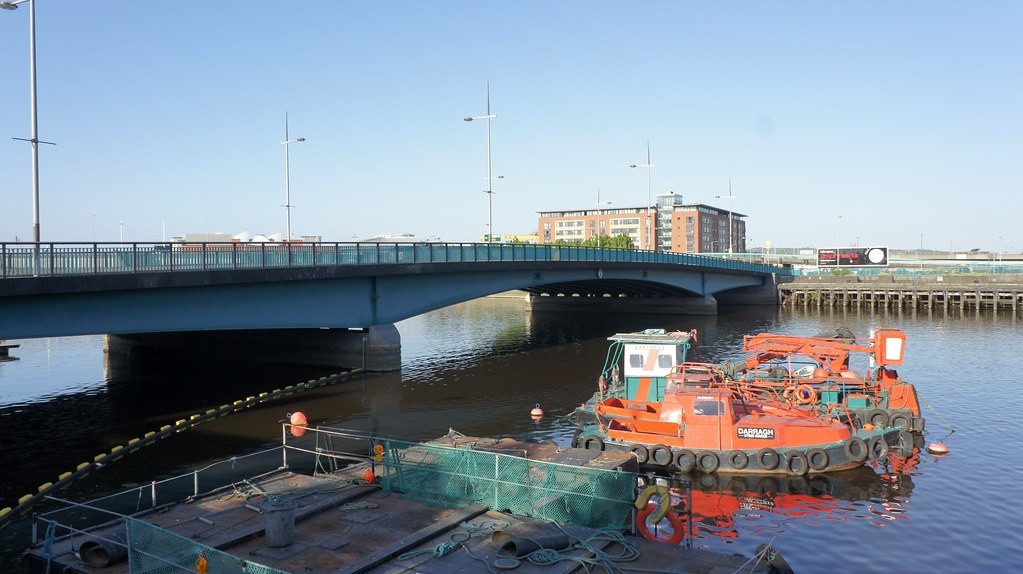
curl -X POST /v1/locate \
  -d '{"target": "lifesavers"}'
[727,450,749,470]
[889,412,911,433]
[867,409,888,429]
[673,449,696,473]
[784,450,808,477]
[648,443,673,468]
[868,436,889,461]
[581,436,604,452]
[570,429,580,447]
[634,484,672,525]
[696,451,720,474]
[844,436,868,463]
[636,504,684,545]
[757,448,779,470]
[783,385,804,405]
[628,444,649,465]
[796,384,815,403]
[806,448,829,471]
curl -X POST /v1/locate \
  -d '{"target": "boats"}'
[728,329,926,432]
[571,327,904,477]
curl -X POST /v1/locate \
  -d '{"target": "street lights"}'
[280,110,306,242]
[715,178,740,259]
[630,141,656,250]
[591,188,611,250]
[0,0,41,275]
[463,79,504,244]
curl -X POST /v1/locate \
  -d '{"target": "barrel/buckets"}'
[261,499,297,547]
